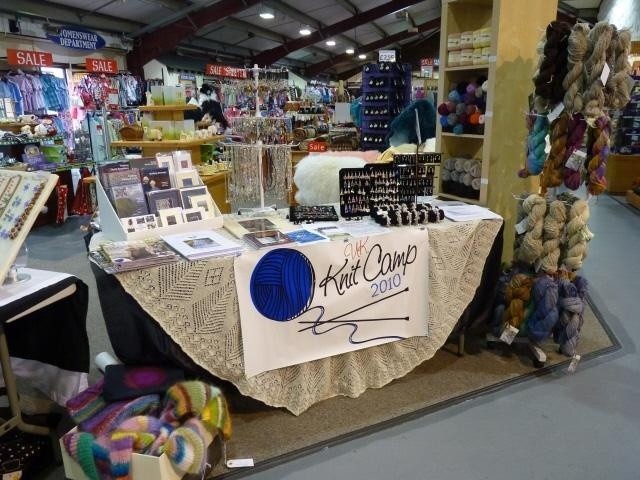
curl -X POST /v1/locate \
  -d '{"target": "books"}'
[101,152,330,272]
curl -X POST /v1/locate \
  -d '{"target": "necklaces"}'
[218,70,296,207]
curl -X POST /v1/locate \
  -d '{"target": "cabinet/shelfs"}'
[109,105,232,215]
[432,0,560,271]
[1,120,76,232]
[360,63,413,151]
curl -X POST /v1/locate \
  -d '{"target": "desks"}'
[2,266,88,436]
[86,195,505,403]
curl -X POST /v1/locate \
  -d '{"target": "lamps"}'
[407,25,419,33]
[326,36,356,55]
[259,5,276,20]
[297,23,312,35]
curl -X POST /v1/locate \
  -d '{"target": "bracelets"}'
[374,204,444,226]
